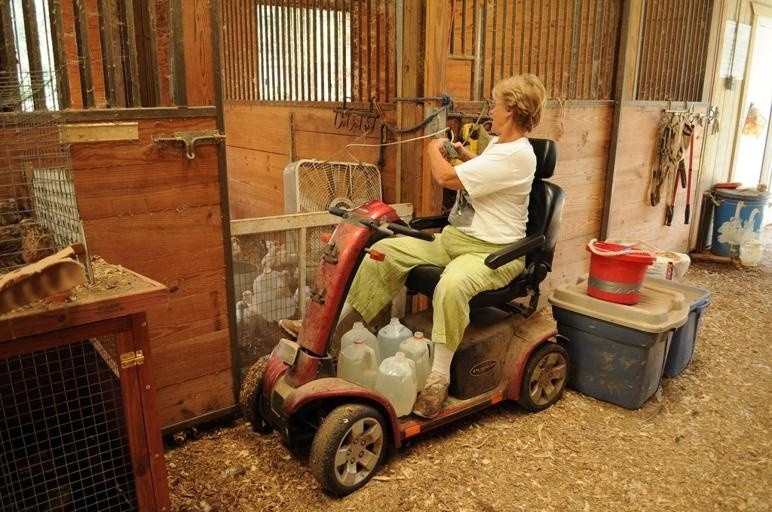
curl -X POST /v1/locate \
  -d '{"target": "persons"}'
[278,72,547,420]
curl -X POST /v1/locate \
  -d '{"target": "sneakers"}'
[414,372,449,419]
[279,318,304,339]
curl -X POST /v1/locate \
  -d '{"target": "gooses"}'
[261,240,313,311]
[230,236,243,263]
[717,201,746,253]
[235,300,250,325]
[242,290,254,324]
[741,208,760,246]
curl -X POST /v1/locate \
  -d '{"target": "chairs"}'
[405,138,566,318]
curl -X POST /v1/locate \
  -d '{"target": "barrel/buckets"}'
[585,238,657,305]
[739,239,764,266]
[252,266,297,323]
[337,339,377,389]
[399,332,433,392]
[336,318,380,368]
[378,318,413,360]
[710,185,772,257]
[376,352,417,419]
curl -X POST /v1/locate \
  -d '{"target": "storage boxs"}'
[640,272,713,381]
[546,280,688,412]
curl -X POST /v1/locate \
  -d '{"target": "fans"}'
[282,158,384,271]
